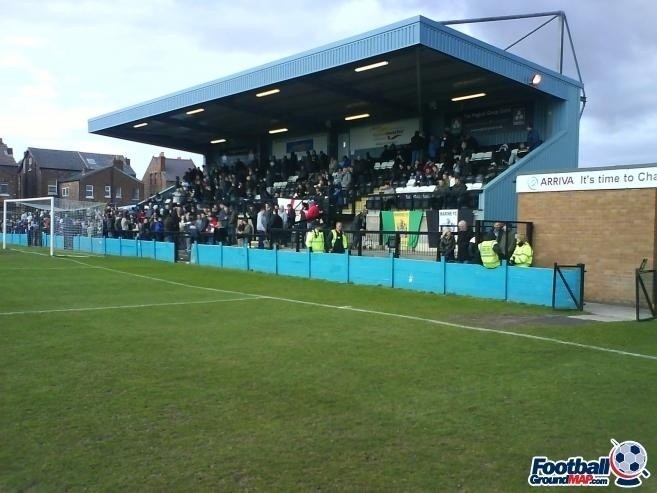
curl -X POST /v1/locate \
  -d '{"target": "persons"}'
[0,199,117,240]
[117,123,541,268]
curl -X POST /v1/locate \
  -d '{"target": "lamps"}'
[530,74,543,86]
